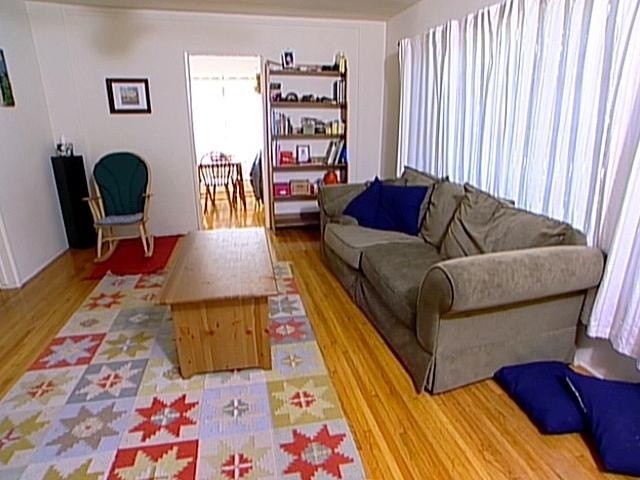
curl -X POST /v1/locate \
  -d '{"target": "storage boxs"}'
[272,180,311,198]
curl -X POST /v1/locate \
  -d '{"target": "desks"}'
[197,161,246,211]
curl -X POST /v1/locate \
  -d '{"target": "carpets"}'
[0,263,365,480]
[80,233,180,279]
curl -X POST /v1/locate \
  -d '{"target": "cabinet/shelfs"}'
[263,58,351,236]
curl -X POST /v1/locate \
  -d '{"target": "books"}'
[269,80,346,197]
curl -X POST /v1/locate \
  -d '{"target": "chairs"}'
[196,151,232,215]
[81,151,155,263]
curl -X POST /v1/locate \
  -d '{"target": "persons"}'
[286,55,292,66]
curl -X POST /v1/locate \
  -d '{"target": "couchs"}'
[310,178,605,396]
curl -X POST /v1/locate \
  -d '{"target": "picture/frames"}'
[297,144,308,163]
[105,77,151,114]
[283,51,295,68]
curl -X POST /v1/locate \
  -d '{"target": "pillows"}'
[491,359,639,477]
[341,177,429,238]
